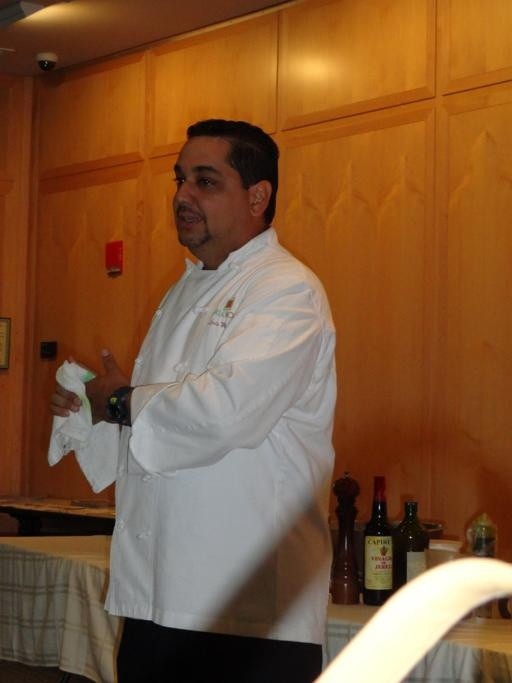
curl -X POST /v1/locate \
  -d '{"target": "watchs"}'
[104,385,134,427]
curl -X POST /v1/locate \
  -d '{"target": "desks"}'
[0,534,512,683]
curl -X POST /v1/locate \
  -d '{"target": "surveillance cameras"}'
[37,52,59,73]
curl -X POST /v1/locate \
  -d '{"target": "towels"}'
[48,361,97,468]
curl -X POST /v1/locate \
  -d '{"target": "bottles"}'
[394,501,430,590]
[471,513,495,618]
[362,474,397,607]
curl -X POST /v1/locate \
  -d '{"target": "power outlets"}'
[39,341,58,361]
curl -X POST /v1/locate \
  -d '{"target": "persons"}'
[47,119,338,682]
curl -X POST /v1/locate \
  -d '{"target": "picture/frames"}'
[1,317,10,368]
